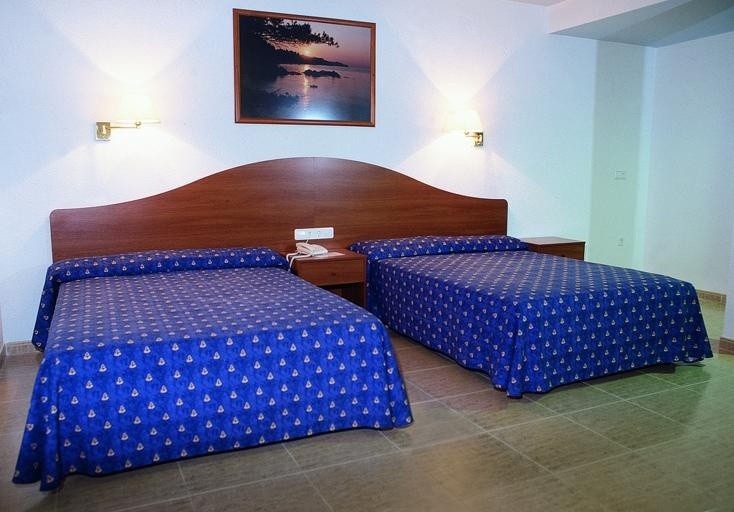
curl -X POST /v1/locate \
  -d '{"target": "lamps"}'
[94,88,162,142]
[443,109,486,149]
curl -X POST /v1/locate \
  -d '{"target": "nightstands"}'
[517,236,585,262]
[278,246,367,311]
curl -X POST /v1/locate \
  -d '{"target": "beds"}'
[44,248,387,480]
[356,232,706,401]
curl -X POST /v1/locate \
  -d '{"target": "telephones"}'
[296,242,328,255]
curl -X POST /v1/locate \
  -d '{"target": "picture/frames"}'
[231,6,377,129]
